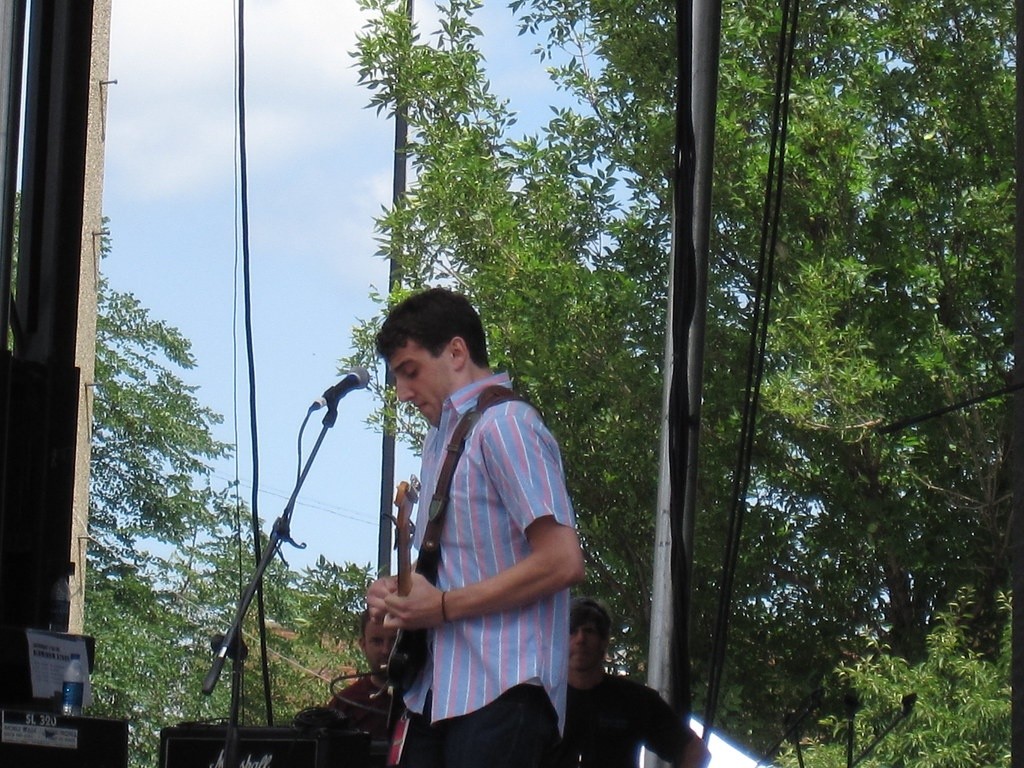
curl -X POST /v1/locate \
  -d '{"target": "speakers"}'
[158,724,372,768]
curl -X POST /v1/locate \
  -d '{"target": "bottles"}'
[63,654,84,717]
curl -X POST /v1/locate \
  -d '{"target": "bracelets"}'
[441,590,448,623]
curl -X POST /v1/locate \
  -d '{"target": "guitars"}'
[385,473,426,736]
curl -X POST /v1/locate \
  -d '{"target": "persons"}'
[367,289,586,768]
[326,598,711,768]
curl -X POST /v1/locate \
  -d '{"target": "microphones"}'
[309,366,371,412]
